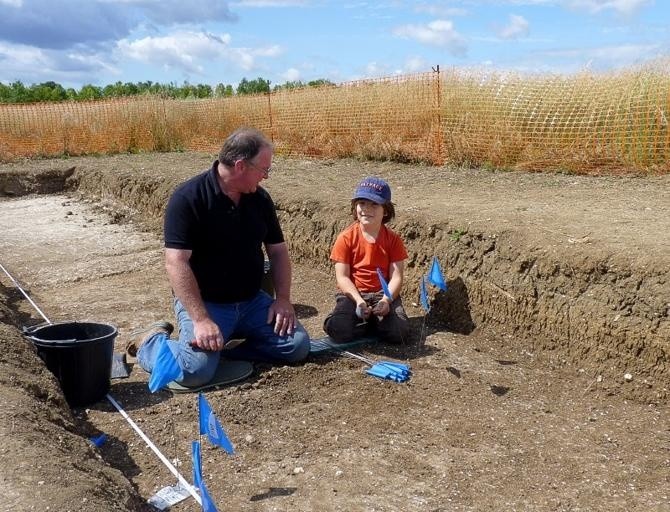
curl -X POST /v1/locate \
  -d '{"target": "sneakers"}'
[124,321,174,358]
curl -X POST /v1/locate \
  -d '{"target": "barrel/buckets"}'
[21,323,117,407]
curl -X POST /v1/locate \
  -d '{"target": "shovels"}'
[356,308,369,327]
[187,339,246,350]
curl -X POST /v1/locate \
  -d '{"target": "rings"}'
[284,317,288,320]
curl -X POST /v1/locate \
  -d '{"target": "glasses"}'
[245,159,272,176]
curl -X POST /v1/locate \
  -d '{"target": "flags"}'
[191,441,218,512]
[428,257,447,291]
[198,393,233,454]
[420,275,430,314]
[377,268,394,301]
[364,360,411,383]
[148,336,182,393]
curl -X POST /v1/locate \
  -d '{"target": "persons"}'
[126,125,311,389]
[322,176,412,346]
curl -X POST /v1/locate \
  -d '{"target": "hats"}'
[351,176,392,205]
